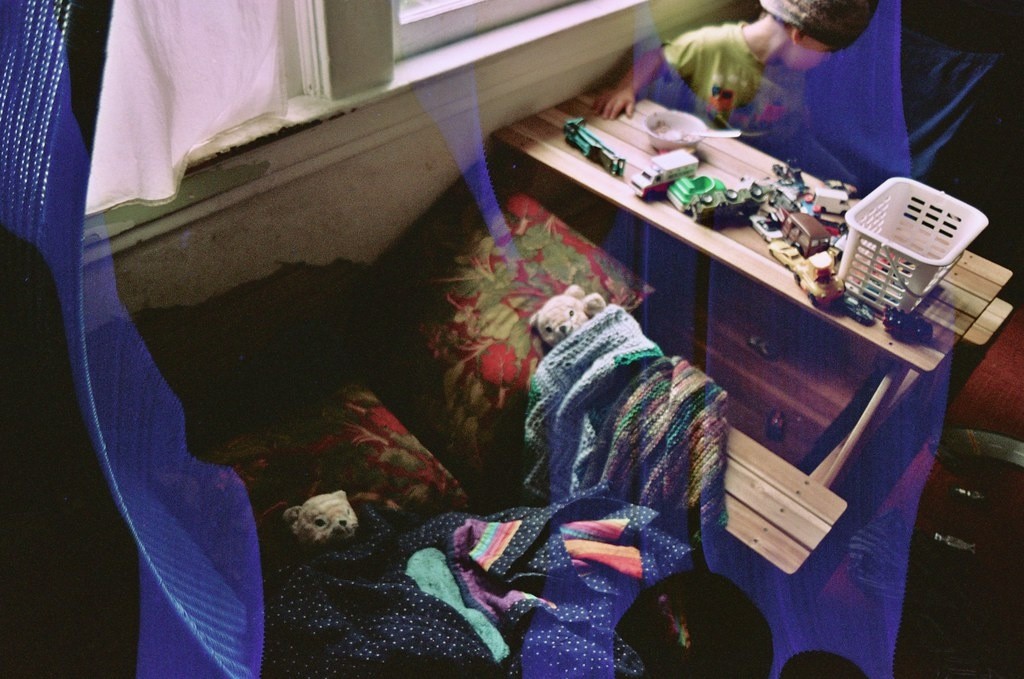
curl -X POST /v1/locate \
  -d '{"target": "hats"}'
[760,0,879,49]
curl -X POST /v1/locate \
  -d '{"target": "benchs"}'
[722,426,848,576]
[946,298,1013,412]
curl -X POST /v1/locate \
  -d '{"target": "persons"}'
[590,0,880,166]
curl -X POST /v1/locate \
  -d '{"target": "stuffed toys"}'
[283,490,359,549]
[527,284,607,347]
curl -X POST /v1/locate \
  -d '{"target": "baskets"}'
[834,176,990,314]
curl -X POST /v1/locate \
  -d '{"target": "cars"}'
[666,173,727,214]
[747,214,784,243]
[766,239,806,272]
[881,303,934,346]
[794,251,847,309]
[841,292,876,327]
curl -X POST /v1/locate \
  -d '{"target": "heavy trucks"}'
[689,181,779,227]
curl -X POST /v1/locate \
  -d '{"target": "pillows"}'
[403,191,657,476]
[193,384,468,571]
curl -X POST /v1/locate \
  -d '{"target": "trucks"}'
[812,186,852,216]
[562,116,626,177]
[631,148,699,202]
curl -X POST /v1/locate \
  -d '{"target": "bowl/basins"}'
[643,110,707,150]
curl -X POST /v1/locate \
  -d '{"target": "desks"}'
[490,84,1014,472]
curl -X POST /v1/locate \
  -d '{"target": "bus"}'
[782,213,832,259]
